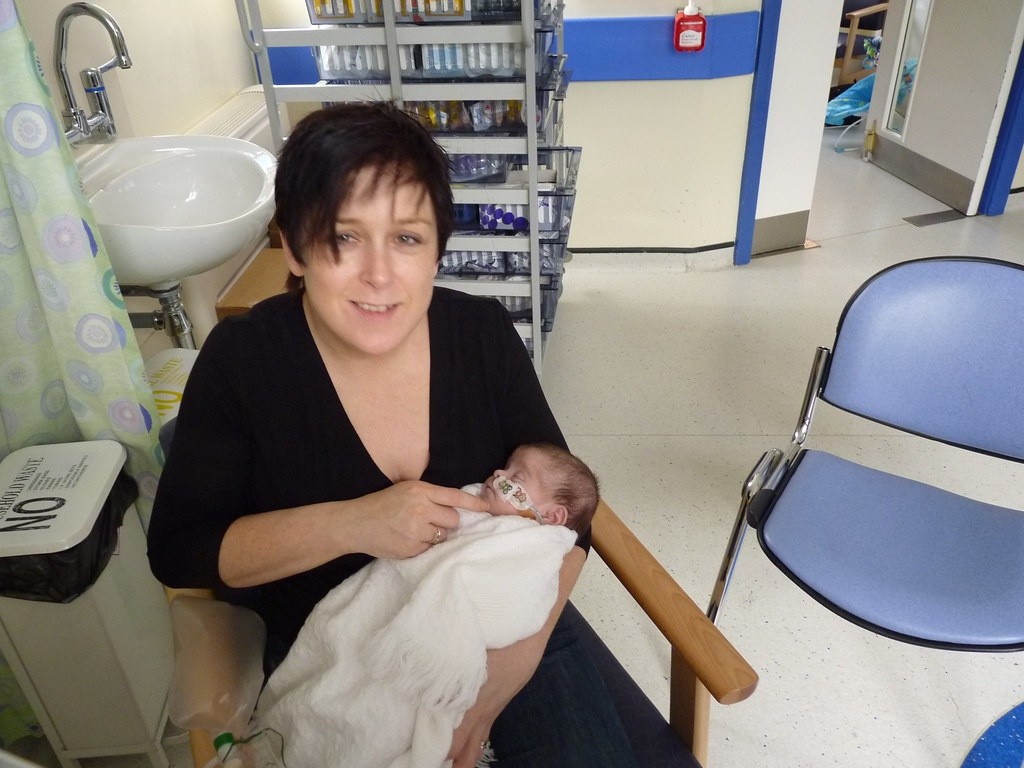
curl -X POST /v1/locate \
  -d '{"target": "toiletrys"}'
[673,0,707,52]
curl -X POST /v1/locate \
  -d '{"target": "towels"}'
[246,481,580,767]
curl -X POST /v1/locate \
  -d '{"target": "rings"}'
[429,528,441,544]
[480,742,487,749]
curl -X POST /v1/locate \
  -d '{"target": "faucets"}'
[51,0,133,142]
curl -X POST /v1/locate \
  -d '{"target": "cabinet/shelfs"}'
[234,0,564,376]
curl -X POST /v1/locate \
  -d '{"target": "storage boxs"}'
[305,0,584,359]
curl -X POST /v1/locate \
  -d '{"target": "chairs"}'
[826,58,920,154]
[156,416,760,768]
[704,254,1024,655]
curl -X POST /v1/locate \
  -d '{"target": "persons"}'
[146,101,639,767]
[227,443,599,768]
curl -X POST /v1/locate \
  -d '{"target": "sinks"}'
[74,137,277,288]
